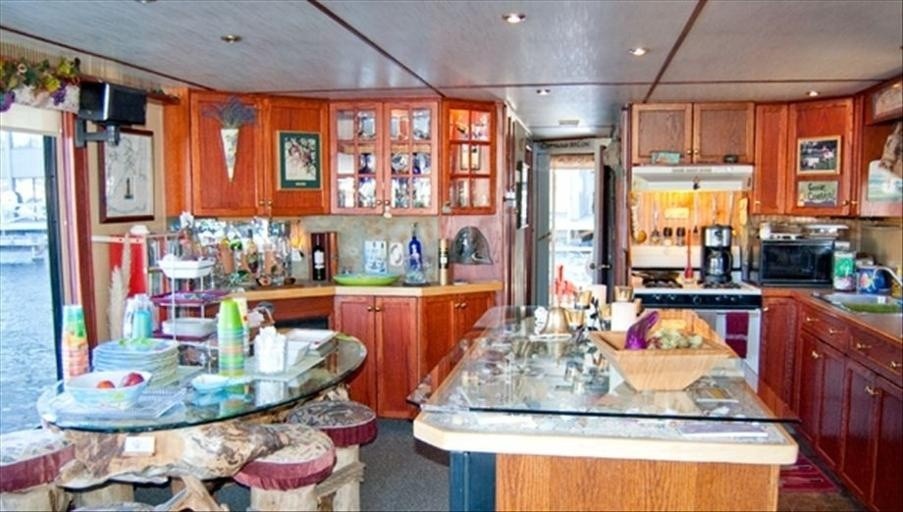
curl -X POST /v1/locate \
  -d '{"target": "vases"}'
[220,126,241,185]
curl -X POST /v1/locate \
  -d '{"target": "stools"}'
[40,419,134,511]
[0,427,79,512]
[280,403,378,510]
[232,421,336,510]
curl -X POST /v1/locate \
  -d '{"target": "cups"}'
[857,266,880,294]
[254,334,287,374]
[216,295,250,379]
[610,302,638,334]
[61,303,90,400]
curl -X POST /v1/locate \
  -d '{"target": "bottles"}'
[647,226,698,247]
[437,238,450,285]
[409,236,423,273]
[758,222,772,241]
[245,228,259,273]
[311,234,327,282]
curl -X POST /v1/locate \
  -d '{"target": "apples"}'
[97,381,115,389]
[123,373,143,388]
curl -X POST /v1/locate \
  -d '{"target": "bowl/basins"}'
[589,330,733,391]
[191,373,228,394]
[723,154,739,162]
[91,338,180,391]
[64,371,152,414]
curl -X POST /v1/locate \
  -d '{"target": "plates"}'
[281,327,341,351]
[331,273,401,286]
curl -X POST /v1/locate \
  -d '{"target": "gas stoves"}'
[631,279,763,310]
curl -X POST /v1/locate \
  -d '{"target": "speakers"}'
[79,80,147,125]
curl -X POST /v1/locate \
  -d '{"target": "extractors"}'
[631,164,754,190]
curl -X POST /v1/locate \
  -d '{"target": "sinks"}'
[812,292,903,314]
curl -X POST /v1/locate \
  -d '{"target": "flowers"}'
[193,93,262,130]
[0,49,80,121]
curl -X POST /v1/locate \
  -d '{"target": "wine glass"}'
[357,151,373,184]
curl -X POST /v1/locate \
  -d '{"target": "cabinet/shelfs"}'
[792,298,852,492]
[162,89,328,219]
[857,74,903,219]
[327,97,441,219]
[332,295,417,421]
[628,100,754,164]
[107,233,194,296]
[788,94,858,219]
[759,290,793,421]
[751,103,787,218]
[440,95,496,217]
[840,322,903,511]
[418,290,497,420]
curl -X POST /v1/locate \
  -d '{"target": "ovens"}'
[695,308,762,376]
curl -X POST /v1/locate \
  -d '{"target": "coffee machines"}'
[699,225,734,286]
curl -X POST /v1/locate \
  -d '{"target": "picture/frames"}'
[793,178,840,211]
[93,123,156,225]
[795,135,842,178]
[273,129,323,194]
[515,157,532,234]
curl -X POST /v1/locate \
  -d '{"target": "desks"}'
[406,304,802,512]
[37,323,368,511]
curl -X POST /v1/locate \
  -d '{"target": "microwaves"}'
[759,239,833,287]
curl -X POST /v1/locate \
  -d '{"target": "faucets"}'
[873,266,903,288]
